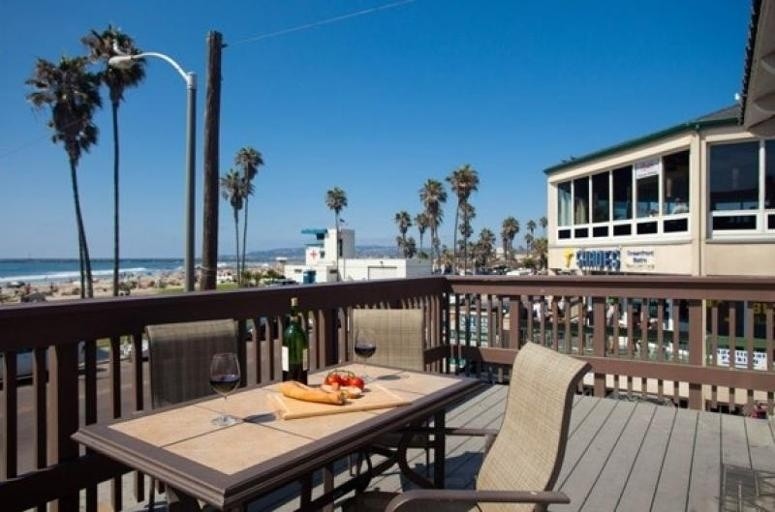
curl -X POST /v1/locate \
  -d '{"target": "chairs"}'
[343,303,429,482]
[142,317,245,511]
[384,339,592,511]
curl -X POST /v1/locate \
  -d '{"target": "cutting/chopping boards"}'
[262,373,412,421]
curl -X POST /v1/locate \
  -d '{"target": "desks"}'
[69,357,490,512]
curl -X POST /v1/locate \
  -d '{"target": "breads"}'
[281,381,343,406]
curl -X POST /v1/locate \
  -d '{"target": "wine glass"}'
[353,327,376,379]
[210,353,242,426]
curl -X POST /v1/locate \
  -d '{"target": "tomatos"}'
[325,371,364,390]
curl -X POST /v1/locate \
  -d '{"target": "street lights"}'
[110,51,198,291]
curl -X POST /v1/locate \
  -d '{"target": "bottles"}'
[282,297,309,341]
[282,311,309,385]
